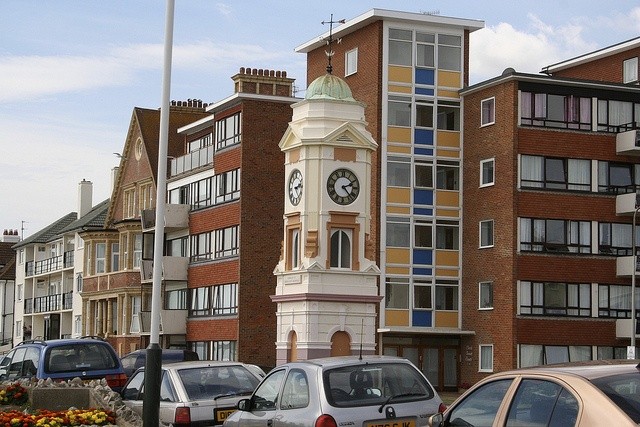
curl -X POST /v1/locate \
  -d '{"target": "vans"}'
[120,349,200,386]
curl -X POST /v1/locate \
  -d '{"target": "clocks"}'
[289,169,302,206]
[327,167,361,206]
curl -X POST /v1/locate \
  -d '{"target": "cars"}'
[1,336,127,393]
[222,319,447,427]
[419,359,640,427]
[115,360,265,427]
